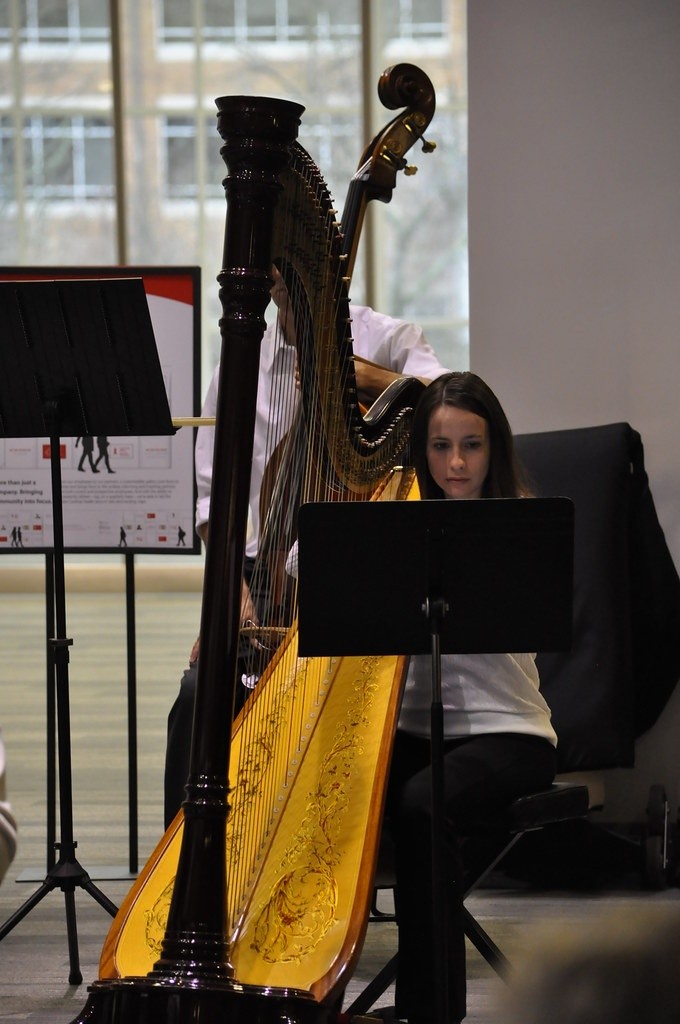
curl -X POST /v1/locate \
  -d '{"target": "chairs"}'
[165,422,680,1024]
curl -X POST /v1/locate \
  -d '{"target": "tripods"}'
[0,276,180,988]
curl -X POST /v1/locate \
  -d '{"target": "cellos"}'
[251,63,437,668]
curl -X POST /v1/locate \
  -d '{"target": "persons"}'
[164,264,450,835]
[285,372,560,1024]
